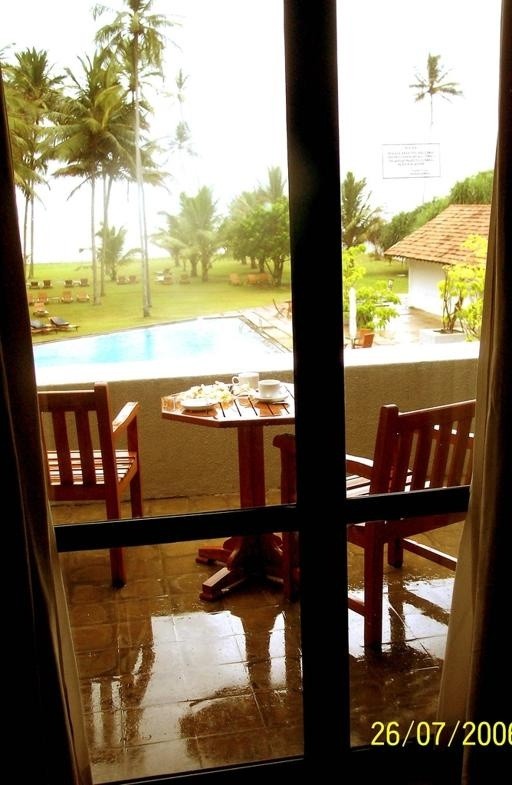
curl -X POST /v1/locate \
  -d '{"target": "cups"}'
[232,372,259,388]
[258,379,287,396]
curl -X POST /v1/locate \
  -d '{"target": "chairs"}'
[272,398,478,667]
[351,327,374,349]
[227,272,270,289]
[35,382,147,590]
[25,278,89,336]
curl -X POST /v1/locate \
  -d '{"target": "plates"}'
[255,396,288,402]
[180,398,218,411]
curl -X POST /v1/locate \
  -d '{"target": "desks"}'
[157,380,295,606]
